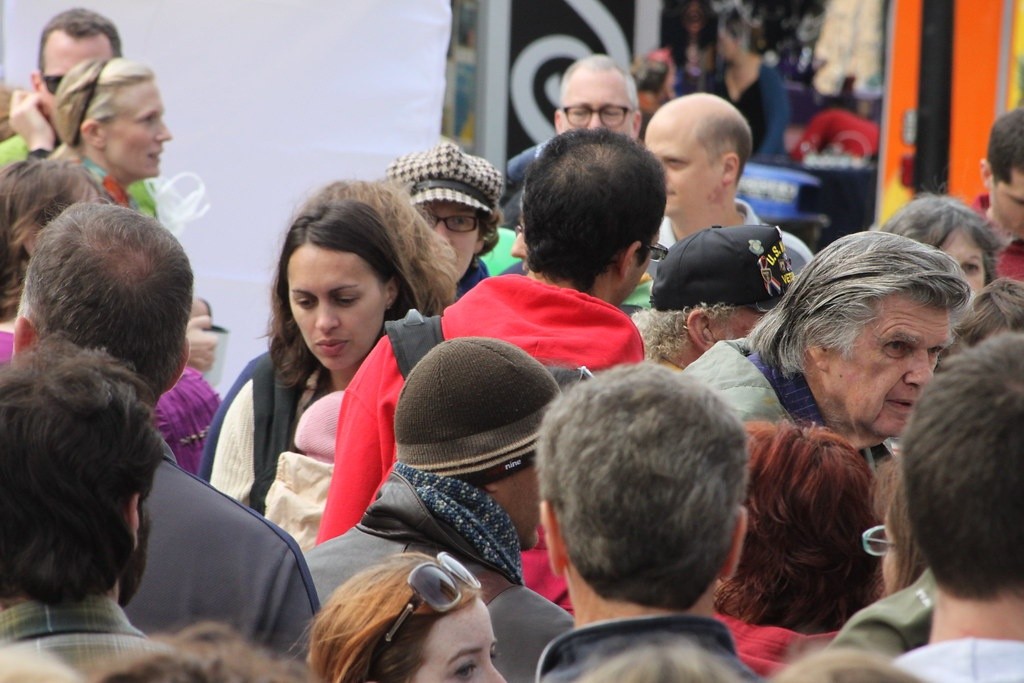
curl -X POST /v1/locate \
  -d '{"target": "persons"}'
[892,330,1024,682]
[535,364,749,683]
[0,335,162,669]
[0,0,1024,683]
[297,335,575,683]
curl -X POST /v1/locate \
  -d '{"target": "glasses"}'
[861,525,895,557]
[625,242,668,261]
[41,71,63,94]
[422,214,480,232]
[559,104,632,128]
[358,551,481,683]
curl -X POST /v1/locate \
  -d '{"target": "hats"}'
[382,143,503,215]
[649,225,795,312]
[393,337,562,487]
